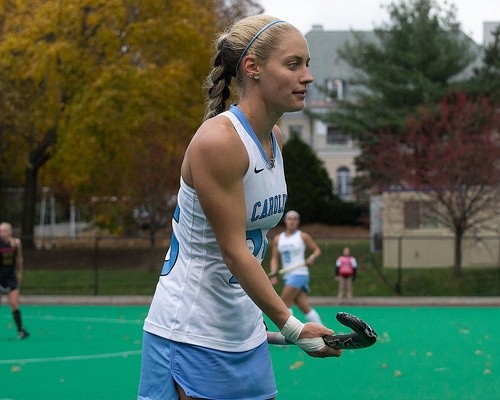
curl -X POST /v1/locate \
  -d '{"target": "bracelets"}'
[311,254,317,261]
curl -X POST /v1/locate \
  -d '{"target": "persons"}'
[333,247,358,305]
[137,16,342,400]
[0,223,31,341]
[268,210,321,328]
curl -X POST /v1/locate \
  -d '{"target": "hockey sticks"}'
[268,311,379,350]
[269,262,306,279]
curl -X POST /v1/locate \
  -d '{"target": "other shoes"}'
[19,330,29,337]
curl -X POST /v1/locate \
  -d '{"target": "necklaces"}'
[238,104,278,168]
